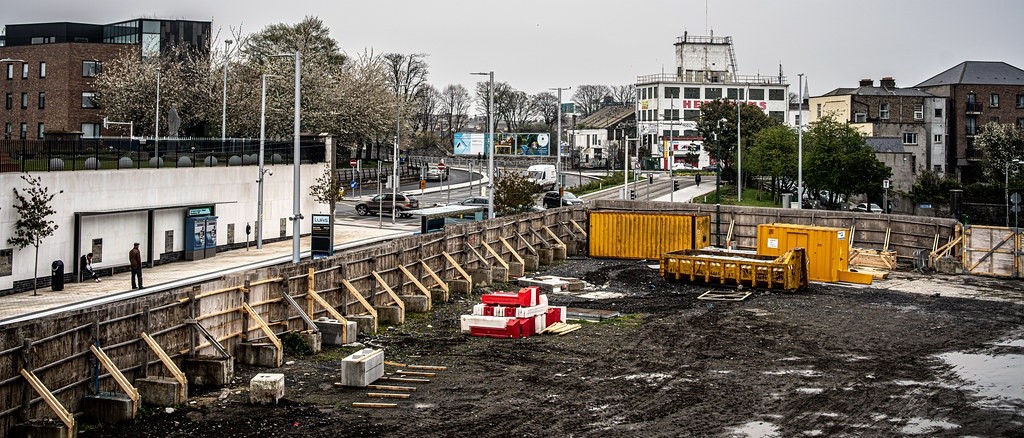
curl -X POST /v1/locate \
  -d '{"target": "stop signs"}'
[351,159,356,167]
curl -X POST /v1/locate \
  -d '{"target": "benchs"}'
[81,261,131,281]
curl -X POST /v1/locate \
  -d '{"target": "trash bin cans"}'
[53,260,64,290]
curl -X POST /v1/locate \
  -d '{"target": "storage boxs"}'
[586,209,714,262]
[756,222,850,283]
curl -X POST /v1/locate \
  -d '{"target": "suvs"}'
[354,192,419,219]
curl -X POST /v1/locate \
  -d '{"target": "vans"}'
[525,164,556,193]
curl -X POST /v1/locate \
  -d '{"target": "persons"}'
[80,253,102,283]
[574,156,579,170]
[695,171,701,187]
[129,242,146,290]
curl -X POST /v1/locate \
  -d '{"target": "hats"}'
[134,243,140,246]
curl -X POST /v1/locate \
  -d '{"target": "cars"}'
[426,166,446,181]
[776,179,842,206]
[543,191,584,210]
[852,202,884,214]
[448,197,496,220]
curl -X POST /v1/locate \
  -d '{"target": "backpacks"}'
[81,255,90,268]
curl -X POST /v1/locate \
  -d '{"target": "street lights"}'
[396,84,407,192]
[623,136,640,199]
[155,67,162,157]
[797,73,805,210]
[222,39,233,150]
[257,74,283,249]
[1005,159,1020,227]
[269,51,304,264]
[470,71,494,219]
[716,118,730,248]
[549,87,572,190]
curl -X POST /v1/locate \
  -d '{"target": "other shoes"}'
[139,287,145,289]
[132,287,138,289]
